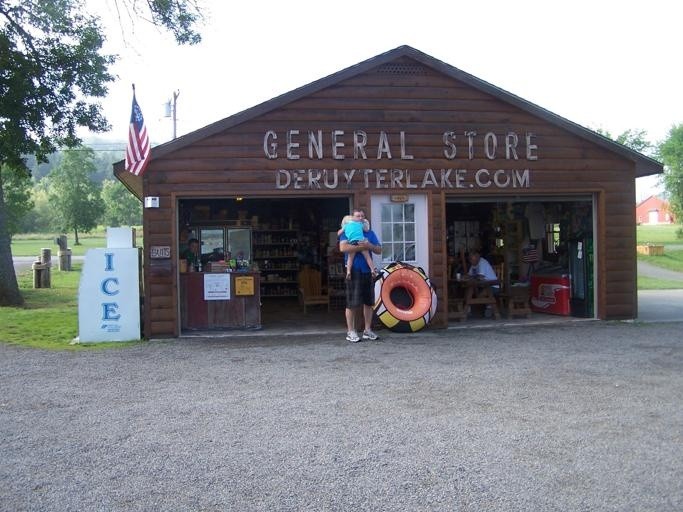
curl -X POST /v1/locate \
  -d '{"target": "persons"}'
[464,251,499,298]
[178,236,198,266]
[335,215,380,283]
[338,207,381,344]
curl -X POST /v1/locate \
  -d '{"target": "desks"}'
[447,279,502,322]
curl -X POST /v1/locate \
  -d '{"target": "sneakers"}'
[345,331,361,343]
[362,329,380,341]
[346,272,352,281]
[371,271,379,281]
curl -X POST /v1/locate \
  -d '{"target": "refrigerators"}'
[529,231,594,318]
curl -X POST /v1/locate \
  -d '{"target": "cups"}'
[456,273,461,280]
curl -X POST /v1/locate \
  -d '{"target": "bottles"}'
[197,260,202,271]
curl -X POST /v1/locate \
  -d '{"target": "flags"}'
[123,93,152,175]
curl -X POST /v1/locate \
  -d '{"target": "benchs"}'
[496,291,532,320]
[448,297,466,321]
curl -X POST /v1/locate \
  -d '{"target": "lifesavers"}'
[372,261,437,333]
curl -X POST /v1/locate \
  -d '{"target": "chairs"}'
[297,264,331,314]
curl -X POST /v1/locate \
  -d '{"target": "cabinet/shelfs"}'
[250,225,344,297]
